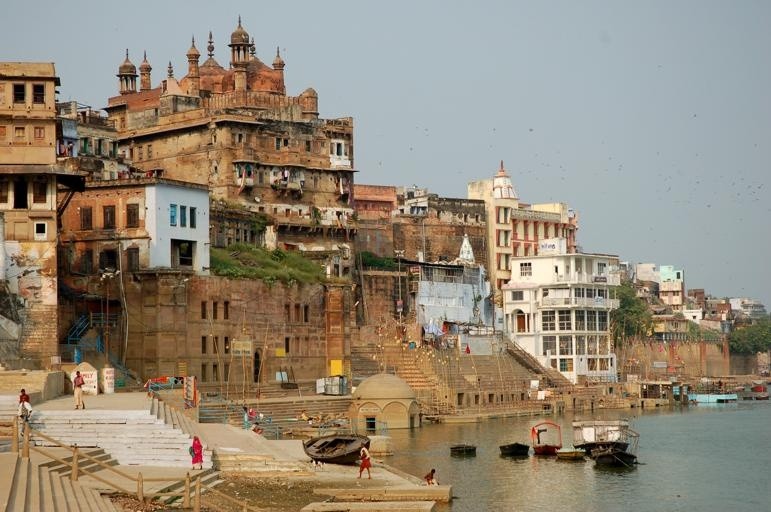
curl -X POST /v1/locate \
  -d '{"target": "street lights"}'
[97,267,121,367]
[393,250,405,324]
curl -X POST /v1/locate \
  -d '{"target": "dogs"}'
[311,459,326,472]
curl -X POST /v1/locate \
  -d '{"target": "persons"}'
[251,422,263,434]
[191,435,203,470]
[352,441,371,480]
[16,396,33,435]
[424,468,436,486]
[300,410,314,422]
[73,370,86,410]
[18,388,29,402]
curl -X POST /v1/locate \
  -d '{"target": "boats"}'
[302,432,371,464]
[500,415,641,468]
[741,392,769,400]
[750,379,768,392]
[450,442,477,453]
